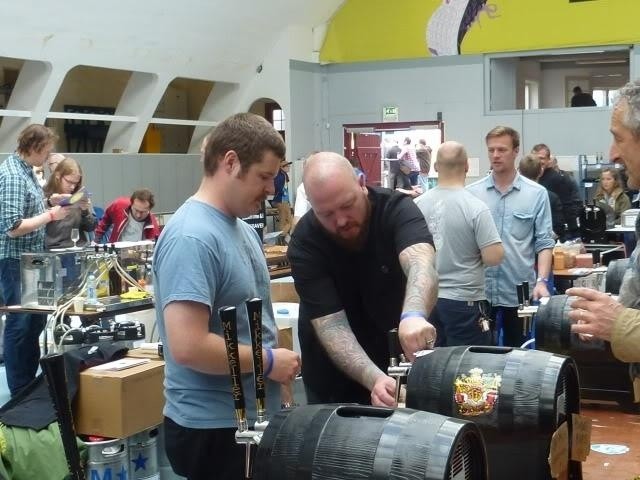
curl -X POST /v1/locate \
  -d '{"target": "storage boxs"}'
[76,355,166,438]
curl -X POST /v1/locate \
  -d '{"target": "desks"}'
[553,259,596,292]
[1,291,156,346]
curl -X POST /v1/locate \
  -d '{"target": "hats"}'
[281,161,292,167]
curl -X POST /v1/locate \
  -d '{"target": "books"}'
[57,185,94,206]
[89,356,148,370]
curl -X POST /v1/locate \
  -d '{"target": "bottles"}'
[87,271,97,305]
[553,238,566,270]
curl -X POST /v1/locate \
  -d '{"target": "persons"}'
[593,166,633,225]
[92,190,160,297]
[269,160,293,244]
[569,82,599,107]
[283,143,447,410]
[291,152,320,237]
[564,75,640,414]
[462,124,557,346]
[518,143,583,244]
[0,122,76,404]
[383,138,432,191]
[32,152,98,305]
[147,109,306,479]
[404,140,510,352]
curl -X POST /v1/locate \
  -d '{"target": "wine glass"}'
[70,228,81,248]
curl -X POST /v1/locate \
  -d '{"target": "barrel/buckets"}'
[606,258,629,294]
[535,294,632,365]
[80,429,187,480]
[405,344,581,438]
[252,402,488,478]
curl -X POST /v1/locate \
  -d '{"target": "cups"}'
[73,296,85,314]
[575,291,611,340]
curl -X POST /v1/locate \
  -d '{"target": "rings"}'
[580,310,585,320]
[426,339,434,345]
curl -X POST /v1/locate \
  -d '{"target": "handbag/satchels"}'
[400,160,411,175]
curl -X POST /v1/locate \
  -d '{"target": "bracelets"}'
[47,195,53,206]
[400,311,427,321]
[48,212,55,223]
